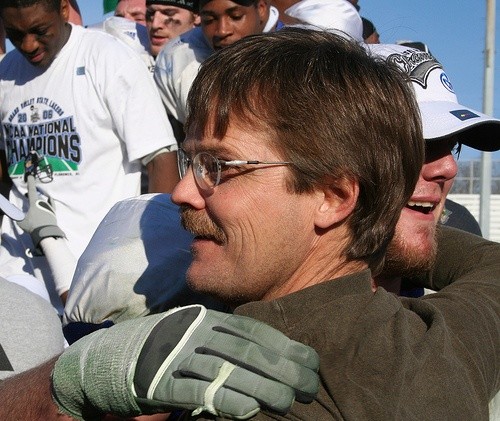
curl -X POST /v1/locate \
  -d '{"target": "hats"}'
[0,194,25,221]
[145,0,198,12]
[357,44,500,154]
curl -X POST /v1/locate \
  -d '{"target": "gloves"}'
[17,175,66,256]
[49,304,320,419]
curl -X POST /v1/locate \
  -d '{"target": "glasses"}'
[177,147,291,190]
